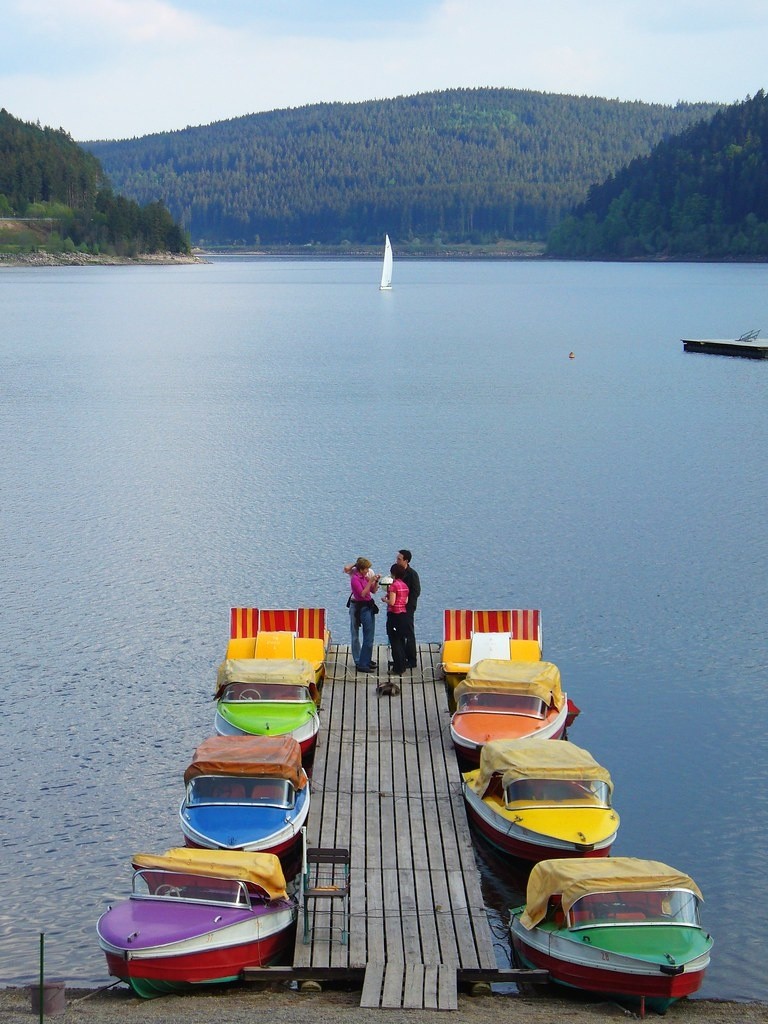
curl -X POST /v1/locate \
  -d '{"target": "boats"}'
[94,845,300,999]
[211,657,320,759]
[459,738,622,866]
[440,606,544,691]
[178,732,311,859]
[508,854,716,1015]
[222,604,331,687]
[446,658,571,766]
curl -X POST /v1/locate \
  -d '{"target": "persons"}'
[381,564,409,675]
[344,550,421,673]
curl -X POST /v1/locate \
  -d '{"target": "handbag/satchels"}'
[346,597,351,607]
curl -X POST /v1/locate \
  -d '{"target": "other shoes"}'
[356,664,358,668]
[389,661,394,665]
[405,664,417,668]
[358,667,374,673]
[370,665,378,669]
[387,669,406,674]
[370,660,376,665]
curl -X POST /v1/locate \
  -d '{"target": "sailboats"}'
[380,232,396,290]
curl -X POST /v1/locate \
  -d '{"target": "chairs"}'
[302,848,350,944]
[251,784,283,799]
[228,631,325,668]
[442,632,541,664]
[212,782,246,799]
[232,684,300,699]
[552,892,662,928]
